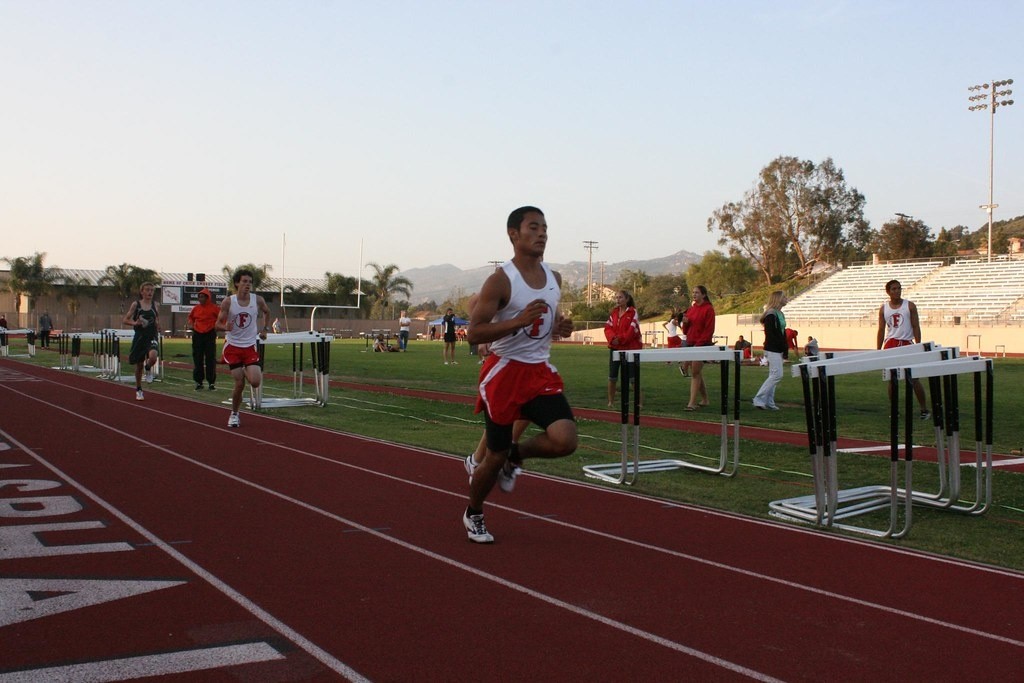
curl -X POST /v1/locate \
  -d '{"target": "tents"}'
[428,316,470,341]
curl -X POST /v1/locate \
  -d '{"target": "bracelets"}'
[264,326,270,331]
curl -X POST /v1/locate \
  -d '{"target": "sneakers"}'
[143,366,153,384]
[135,390,144,400]
[499,441,523,492]
[227,411,240,427]
[462,506,495,543]
[463,454,481,485]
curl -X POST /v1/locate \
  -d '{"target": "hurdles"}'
[0,328,36,358]
[749,327,766,357]
[966,334,982,357]
[767,340,995,538]
[583,336,594,345]
[53,328,165,383]
[361,328,391,352]
[581,344,746,487]
[711,336,728,346]
[995,344,1006,358]
[641,330,665,350]
[221,329,335,410]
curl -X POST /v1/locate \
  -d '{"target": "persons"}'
[399,311,411,351]
[373,333,404,352]
[463,207,577,544]
[123,282,162,400]
[0,315,7,348]
[663,285,720,412]
[735,335,752,359]
[187,288,227,392]
[752,291,788,410]
[214,270,271,428]
[604,290,645,410]
[804,336,818,356]
[877,280,931,421]
[430,325,468,344]
[272,317,283,334]
[39,309,53,350]
[443,308,459,364]
[783,328,798,363]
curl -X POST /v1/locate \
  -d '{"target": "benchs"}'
[781,258,1024,328]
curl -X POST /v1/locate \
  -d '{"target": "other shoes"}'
[919,410,932,420]
[445,362,448,364]
[765,404,779,411]
[683,405,700,411]
[696,403,709,407]
[193,384,204,392]
[758,406,766,410]
[478,361,483,365]
[209,384,217,391]
[451,361,459,364]
[888,413,900,418]
